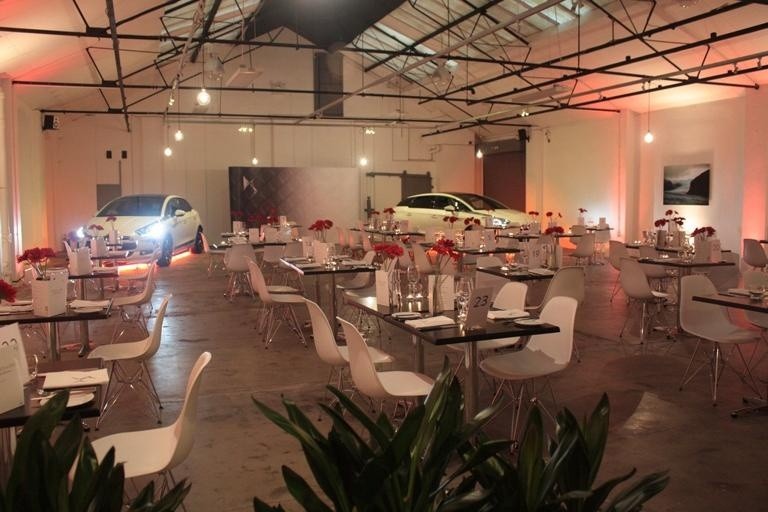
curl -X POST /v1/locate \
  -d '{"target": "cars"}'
[390,192,537,242]
[75,194,205,267]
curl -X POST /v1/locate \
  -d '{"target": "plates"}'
[73,308,103,314]
[34,391,95,407]
[513,317,545,326]
[392,311,421,319]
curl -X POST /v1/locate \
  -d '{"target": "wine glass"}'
[408,264,421,298]
[234,224,241,241]
[333,246,342,261]
[325,246,335,267]
[240,222,249,238]
[456,281,470,319]
[460,276,475,312]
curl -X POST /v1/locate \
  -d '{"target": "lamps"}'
[173,73,184,141]
[360,127,367,167]
[252,124,258,166]
[476,123,484,159]
[164,108,173,158]
[197,9,212,108]
[644,81,654,143]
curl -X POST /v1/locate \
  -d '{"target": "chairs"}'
[480,296,578,455]
[87,296,169,430]
[680,275,762,406]
[447,281,528,396]
[68,351,213,511]
[336,316,435,439]
[303,298,396,420]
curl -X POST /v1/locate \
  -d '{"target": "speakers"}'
[44,115,53,128]
[519,129,526,140]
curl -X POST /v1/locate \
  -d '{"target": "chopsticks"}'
[30,390,90,401]
